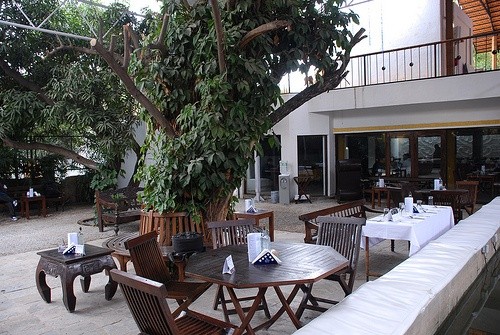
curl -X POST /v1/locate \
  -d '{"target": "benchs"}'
[0,178,64,212]
[96,186,146,236]
[298,197,391,262]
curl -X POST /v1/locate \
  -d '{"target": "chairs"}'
[110,268,239,335]
[295,216,367,321]
[207,218,271,331]
[408,181,479,225]
[123,230,214,319]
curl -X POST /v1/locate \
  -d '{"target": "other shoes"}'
[10,216,17,221]
[13,200,17,207]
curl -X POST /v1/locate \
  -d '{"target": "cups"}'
[384,208,389,216]
[399,202,404,212]
[428,196,433,207]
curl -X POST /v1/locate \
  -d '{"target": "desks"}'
[233,210,274,243]
[465,169,500,200]
[184,242,350,335]
[371,186,403,210]
[35,244,118,312]
[352,204,455,283]
[393,169,407,178]
[20,193,46,218]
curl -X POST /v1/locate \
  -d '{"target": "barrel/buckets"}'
[270,191,279,204]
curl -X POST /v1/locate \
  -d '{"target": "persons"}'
[402,153,411,176]
[433,144,440,158]
[0,183,17,221]
[262,158,273,181]
[373,155,386,176]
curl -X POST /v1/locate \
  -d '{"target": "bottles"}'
[260,229,271,251]
[254,198,258,213]
[77,227,84,245]
[438,177,443,190]
[279,161,288,174]
